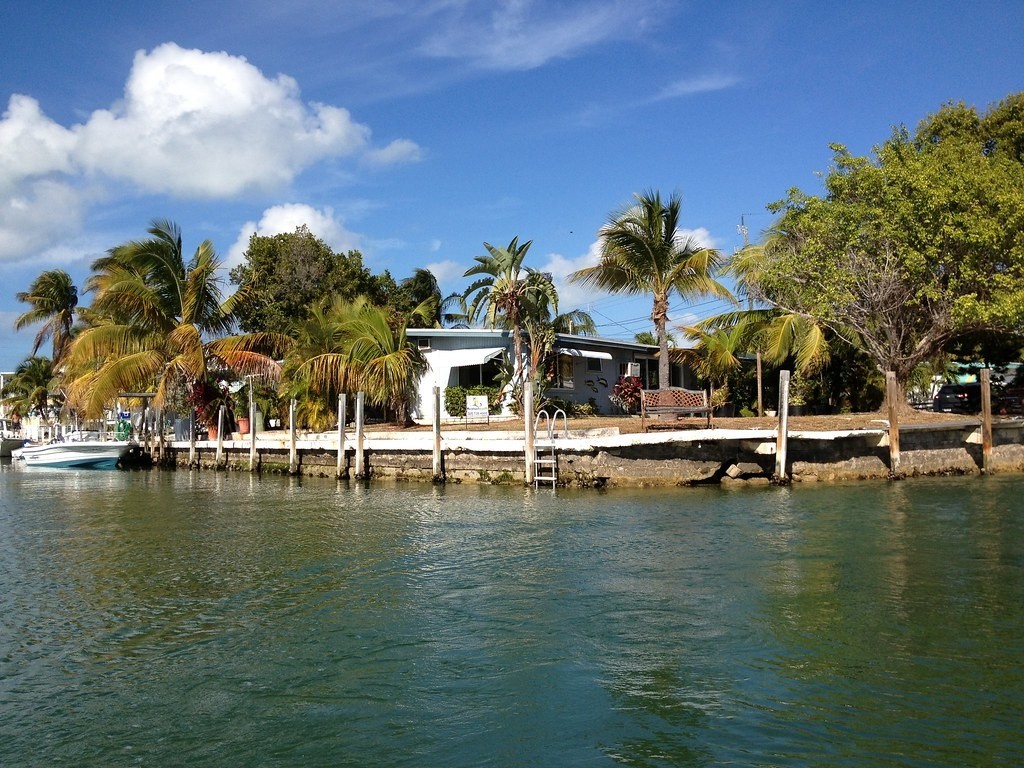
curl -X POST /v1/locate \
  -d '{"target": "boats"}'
[10,425,136,472]
[0,419,26,457]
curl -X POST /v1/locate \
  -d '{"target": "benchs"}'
[640,387,716,432]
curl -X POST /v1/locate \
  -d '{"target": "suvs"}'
[932,383,1024,415]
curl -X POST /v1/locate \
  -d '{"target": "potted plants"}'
[788,395,807,415]
